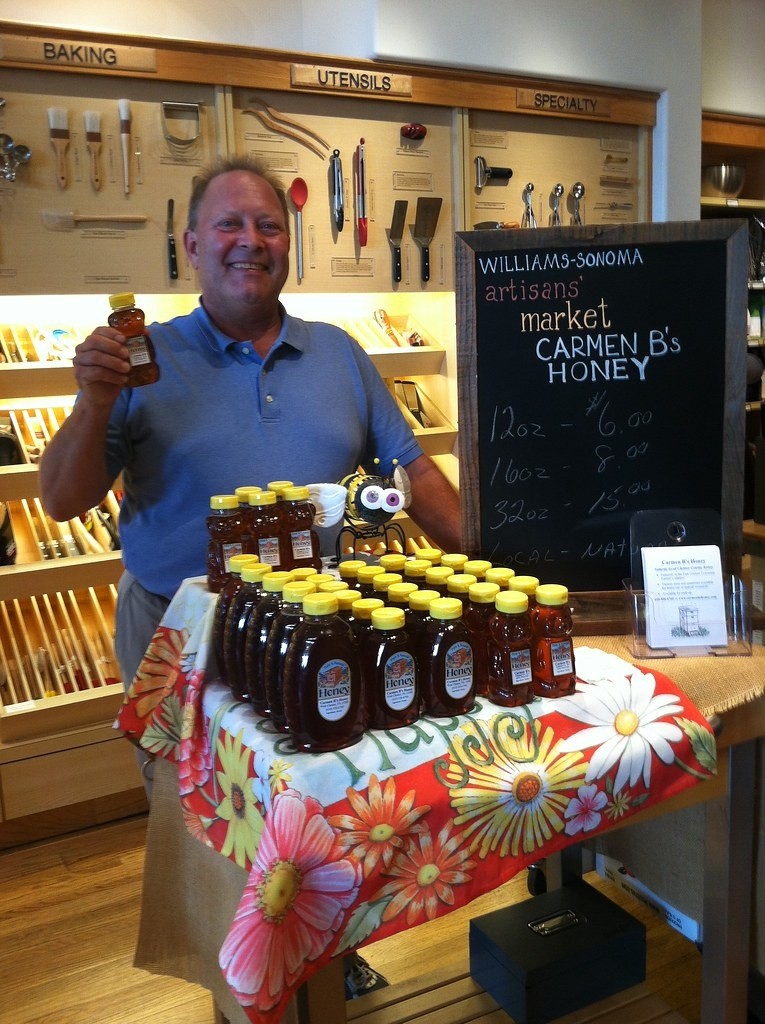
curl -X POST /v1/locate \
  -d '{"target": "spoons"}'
[290,176,316,282]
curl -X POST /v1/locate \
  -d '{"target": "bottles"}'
[205,478,577,753]
[106,291,164,390]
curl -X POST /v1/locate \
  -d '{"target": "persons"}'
[37,155,465,809]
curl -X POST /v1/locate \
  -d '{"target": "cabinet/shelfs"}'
[0,311,461,852]
[699,109,765,411]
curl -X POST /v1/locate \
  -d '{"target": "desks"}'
[193,626,765,1023]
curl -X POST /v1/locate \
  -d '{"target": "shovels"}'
[166,198,180,278]
[415,197,443,282]
[388,200,409,282]
[472,222,522,230]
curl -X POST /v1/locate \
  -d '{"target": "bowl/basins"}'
[701,163,746,198]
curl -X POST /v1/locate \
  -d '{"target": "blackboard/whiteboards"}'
[445,216,750,604]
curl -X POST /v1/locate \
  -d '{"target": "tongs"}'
[355,137,368,246]
[330,148,348,235]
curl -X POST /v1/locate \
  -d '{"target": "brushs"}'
[46,105,72,188]
[118,97,131,194]
[84,110,106,191]
[38,208,148,229]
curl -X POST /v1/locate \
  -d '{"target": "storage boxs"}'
[469,879,646,1024]
